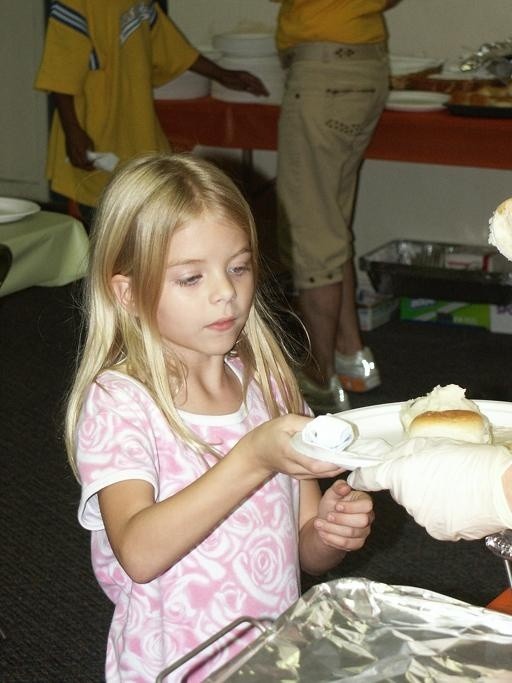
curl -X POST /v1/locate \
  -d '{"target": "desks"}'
[0,211,91,296]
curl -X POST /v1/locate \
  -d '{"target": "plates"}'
[378,56,441,76]
[288,398,512,469]
[385,88,450,112]
[2,194,43,223]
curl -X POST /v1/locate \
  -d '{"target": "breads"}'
[485,196,512,263]
[397,379,495,441]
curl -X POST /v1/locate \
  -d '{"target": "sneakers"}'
[291,348,380,417]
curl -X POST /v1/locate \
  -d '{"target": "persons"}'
[271,0,401,414]
[64,154,377,683]
[35,0,269,343]
